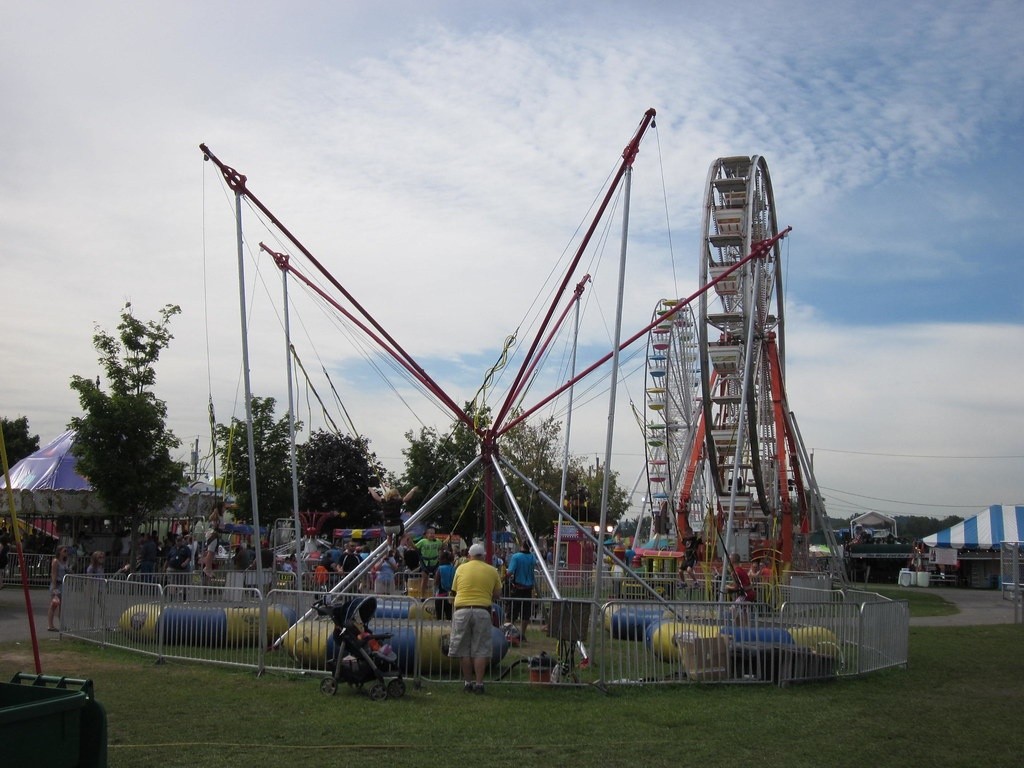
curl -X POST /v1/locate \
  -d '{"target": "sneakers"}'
[462,681,485,693]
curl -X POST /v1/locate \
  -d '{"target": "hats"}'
[467,544,486,556]
[174,536,183,540]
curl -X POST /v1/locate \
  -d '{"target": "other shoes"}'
[47,625,69,632]
[518,633,527,642]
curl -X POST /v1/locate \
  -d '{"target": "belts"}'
[454,603,486,609]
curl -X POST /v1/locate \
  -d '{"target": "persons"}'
[87,552,111,633]
[282,557,292,572]
[46,546,71,631]
[409,528,448,602]
[202,501,226,578]
[933,551,936,561]
[750,559,784,586]
[724,551,756,629]
[163,535,191,602]
[290,554,298,570]
[678,527,702,588]
[314,558,328,600]
[373,547,398,597]
[3,527,196,571]
[326,523,614,573]
[0,529,9,589]
[434,551,454,622]
[350,618,391,656]
[843,529,908,548]
[930,550,934,562]
[505,539,535,641]
[253,541,274,570]
[368,485,418,550]
[232,544,257,569]
[450,544,502,695]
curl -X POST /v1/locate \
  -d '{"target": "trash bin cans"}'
[0,670,108,768]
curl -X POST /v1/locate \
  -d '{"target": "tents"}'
[923,503,1024,599]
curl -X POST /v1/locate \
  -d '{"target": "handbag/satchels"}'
[50,589,59,610]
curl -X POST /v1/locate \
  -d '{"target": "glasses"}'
[60,548,67,554]
[350,545,356,548]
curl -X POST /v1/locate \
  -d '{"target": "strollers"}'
[309,592,408,703]
